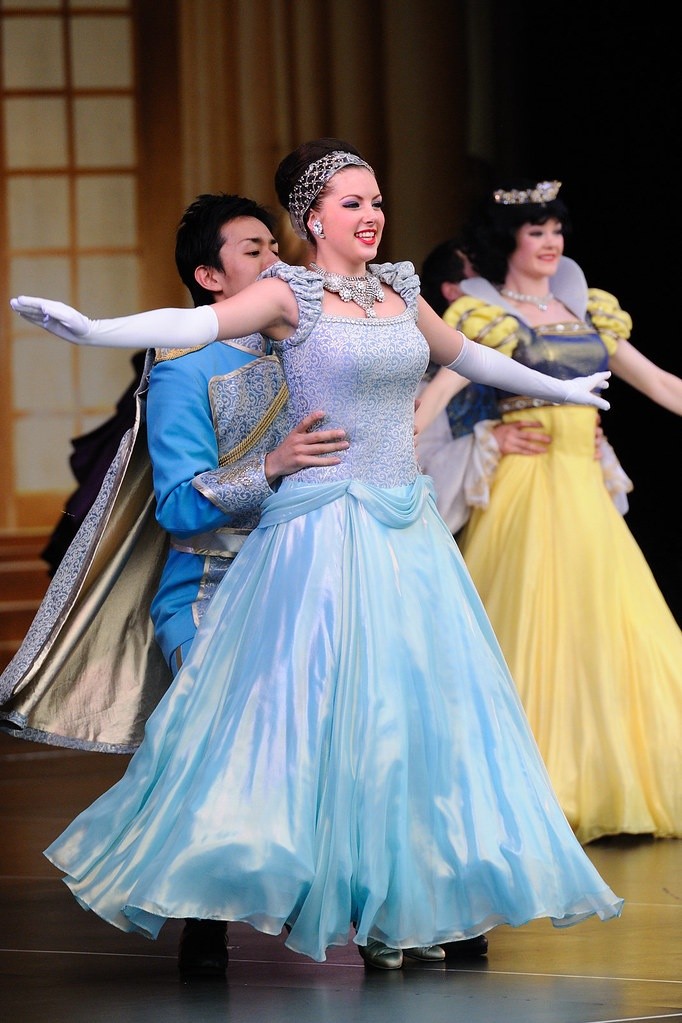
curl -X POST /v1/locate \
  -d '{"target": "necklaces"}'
[310,260,385,320]
[501,287,553,310]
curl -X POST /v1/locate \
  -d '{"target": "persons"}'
[10,138,612,974]
[0,189,488,973]
[411,171,682,849]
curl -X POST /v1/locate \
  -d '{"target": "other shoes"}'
[438,934,488,959]
[178,918,230,977]
[401,945,446,962]
[352,921,404,970]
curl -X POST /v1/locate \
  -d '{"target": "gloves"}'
[441,329,611,412]
[8,294,220,350]
